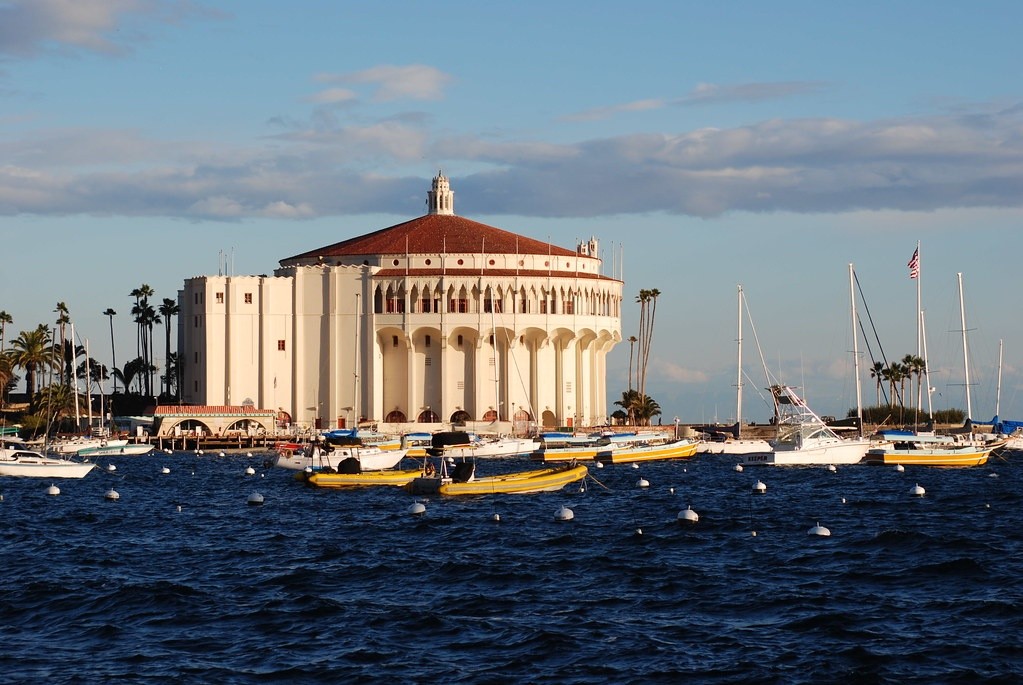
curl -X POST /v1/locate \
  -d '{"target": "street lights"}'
[424,404,434,423]
[497,401,504,420]
[103,308,118,392]
[673,416,680,441]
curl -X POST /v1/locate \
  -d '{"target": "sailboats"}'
[274,232,1023,474]
[0,322,156,456]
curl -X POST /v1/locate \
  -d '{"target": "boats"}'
[305,423,440,488]
[410,430,589,497]
[0,450,98,479]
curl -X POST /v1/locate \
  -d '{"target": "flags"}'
[908,246,918,278]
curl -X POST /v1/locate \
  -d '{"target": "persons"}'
[658,417,662,426]
